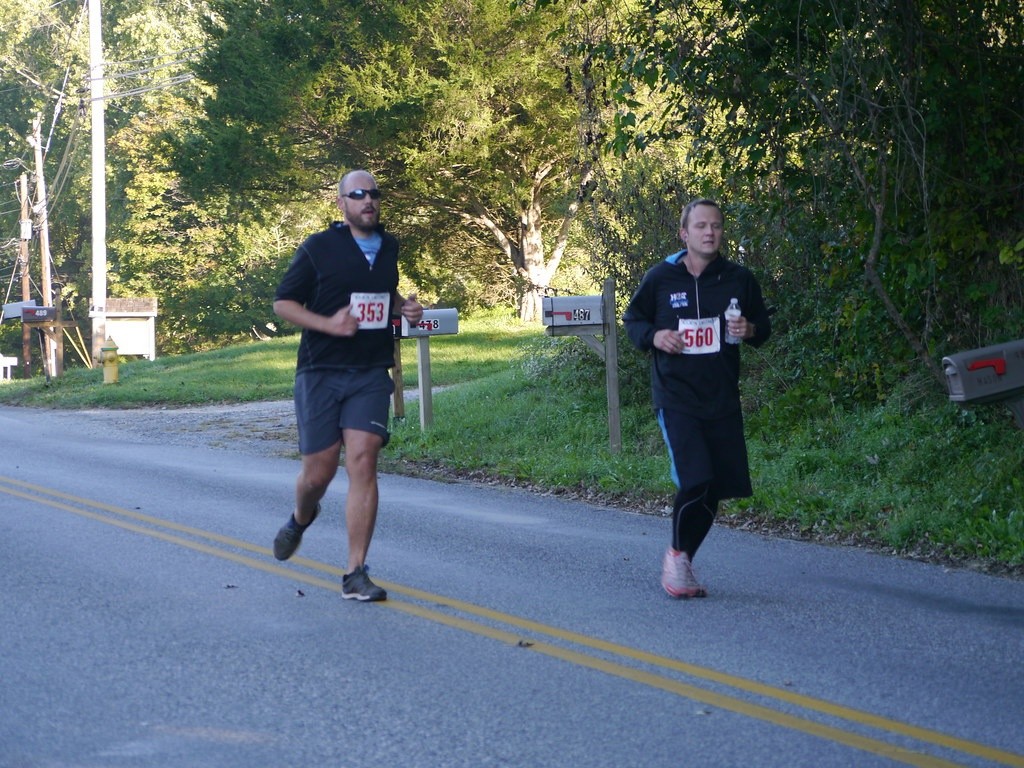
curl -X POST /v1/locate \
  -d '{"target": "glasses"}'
[343,189,381,200]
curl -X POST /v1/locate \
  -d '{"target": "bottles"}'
[724,298,742,344]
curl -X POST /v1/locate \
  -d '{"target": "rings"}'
[737,329,739,334]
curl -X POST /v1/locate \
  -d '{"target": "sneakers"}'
[342,564,388,602]
[661,543,706,598]
[273,501,321,561]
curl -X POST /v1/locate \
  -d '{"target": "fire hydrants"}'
[93,336,120,385]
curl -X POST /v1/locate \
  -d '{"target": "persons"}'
[622,200,771,600]
[272,172,422,603]
